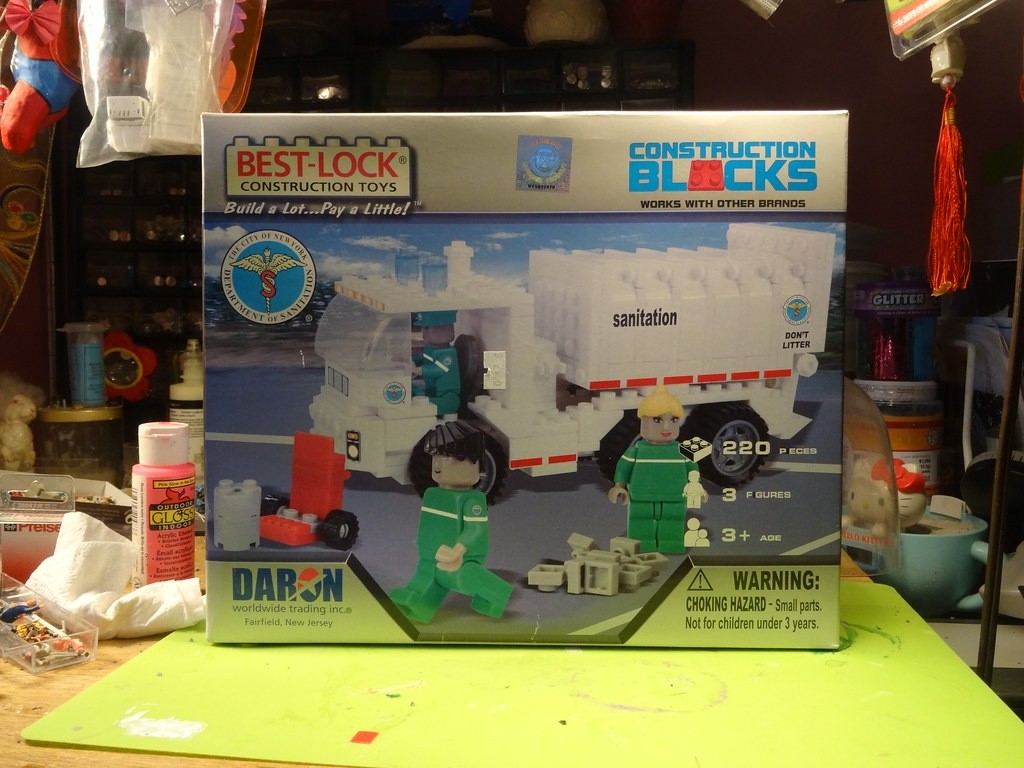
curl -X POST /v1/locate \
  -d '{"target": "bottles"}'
[854,380,940,496]
[62,323,107,409]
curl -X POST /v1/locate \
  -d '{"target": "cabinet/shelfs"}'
[62,37,698,440]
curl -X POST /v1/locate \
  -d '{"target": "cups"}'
[849,506,1007,618]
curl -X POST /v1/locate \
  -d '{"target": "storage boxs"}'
[0,570,99,674]
[196,112,848,651]
[0,471,137,585]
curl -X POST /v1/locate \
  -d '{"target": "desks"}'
[0,534,876,768]
